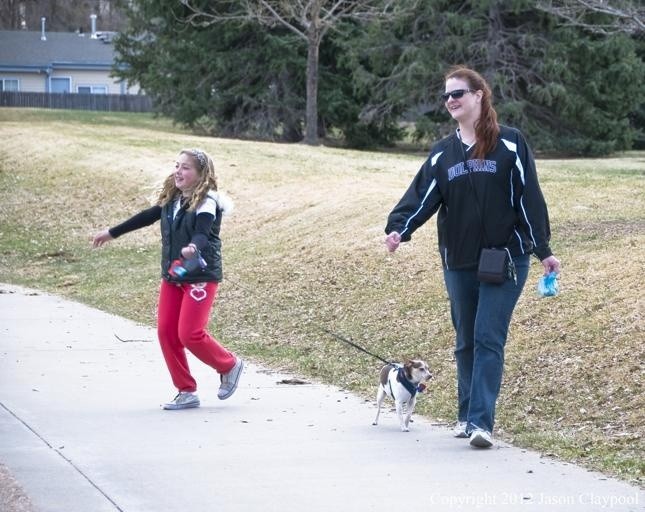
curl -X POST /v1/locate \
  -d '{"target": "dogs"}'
[372,358,433,433]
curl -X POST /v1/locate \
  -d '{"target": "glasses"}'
[440,90,477,102]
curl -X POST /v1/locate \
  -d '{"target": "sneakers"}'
[162,392,200,411]
[217,357,243,399]
[453,422,469,438]
[468,429,493,448]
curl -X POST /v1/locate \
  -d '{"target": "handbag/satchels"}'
[477,247,509,283]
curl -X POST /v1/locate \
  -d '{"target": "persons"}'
[384,66,563,448]
[92,146,244,411]
